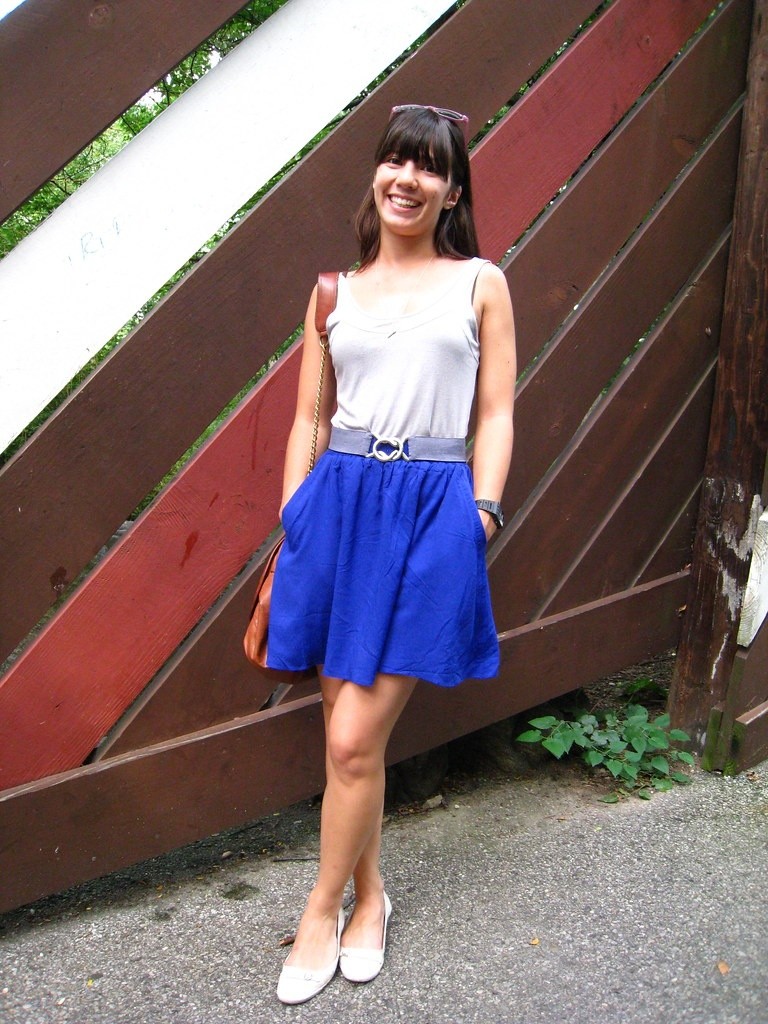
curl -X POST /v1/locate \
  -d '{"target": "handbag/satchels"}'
[242,537,316,683]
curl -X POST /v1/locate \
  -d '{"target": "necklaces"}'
[374,246,441,339]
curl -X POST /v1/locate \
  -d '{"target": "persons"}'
[272,101,517,1007]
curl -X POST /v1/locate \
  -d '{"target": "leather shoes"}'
[278,891,392,1005]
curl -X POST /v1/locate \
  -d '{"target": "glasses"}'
[388,104,470,150]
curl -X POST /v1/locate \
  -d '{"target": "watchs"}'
[472,498,505,529]
